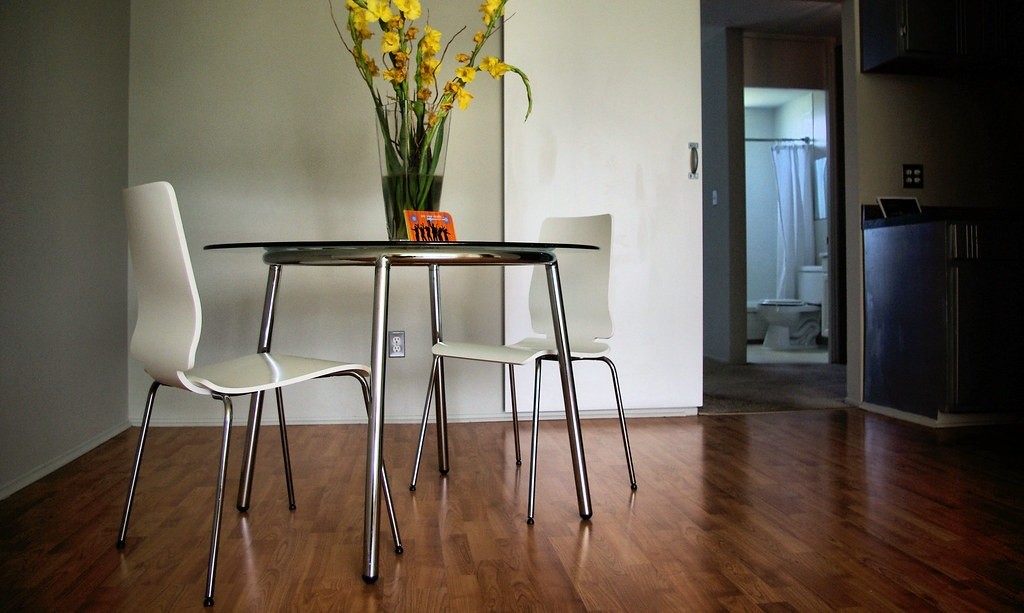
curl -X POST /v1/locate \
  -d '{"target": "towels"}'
[815,157,827,221]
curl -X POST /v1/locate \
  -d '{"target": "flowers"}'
[329,0,532,122]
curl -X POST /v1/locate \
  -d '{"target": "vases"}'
[375,101,451,240]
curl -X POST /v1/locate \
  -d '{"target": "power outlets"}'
[388,330,404,357]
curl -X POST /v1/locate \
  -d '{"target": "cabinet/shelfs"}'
[859,0,1023,73]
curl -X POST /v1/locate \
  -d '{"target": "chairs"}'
[116,180,403,605]
[410,213,636,525]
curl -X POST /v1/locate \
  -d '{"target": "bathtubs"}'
[747,302,758,340]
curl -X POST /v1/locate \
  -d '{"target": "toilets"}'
[757,266,823,352]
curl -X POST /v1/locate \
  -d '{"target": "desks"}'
[202,241,600,585]
